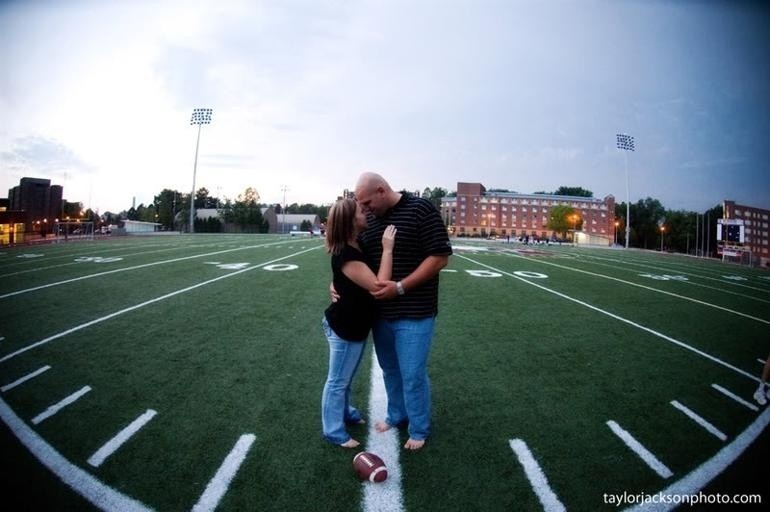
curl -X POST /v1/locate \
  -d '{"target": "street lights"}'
[615,132,637,250]
[216,185,223,208]
[280,184,288,234]
[567,214,580,231]
[186,106,214,233]
[660,226,666,252]
[615,221,620,244]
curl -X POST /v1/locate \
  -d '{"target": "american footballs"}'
[353,452,388,483]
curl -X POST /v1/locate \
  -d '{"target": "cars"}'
[71,224,119,235]
[311,222,326,236]
[489,233,496,240]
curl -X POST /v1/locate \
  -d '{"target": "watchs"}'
[397,280,405,296]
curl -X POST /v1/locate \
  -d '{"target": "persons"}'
[329,169,454,450]
[506,232,564,248]
[319,197,399,450]
